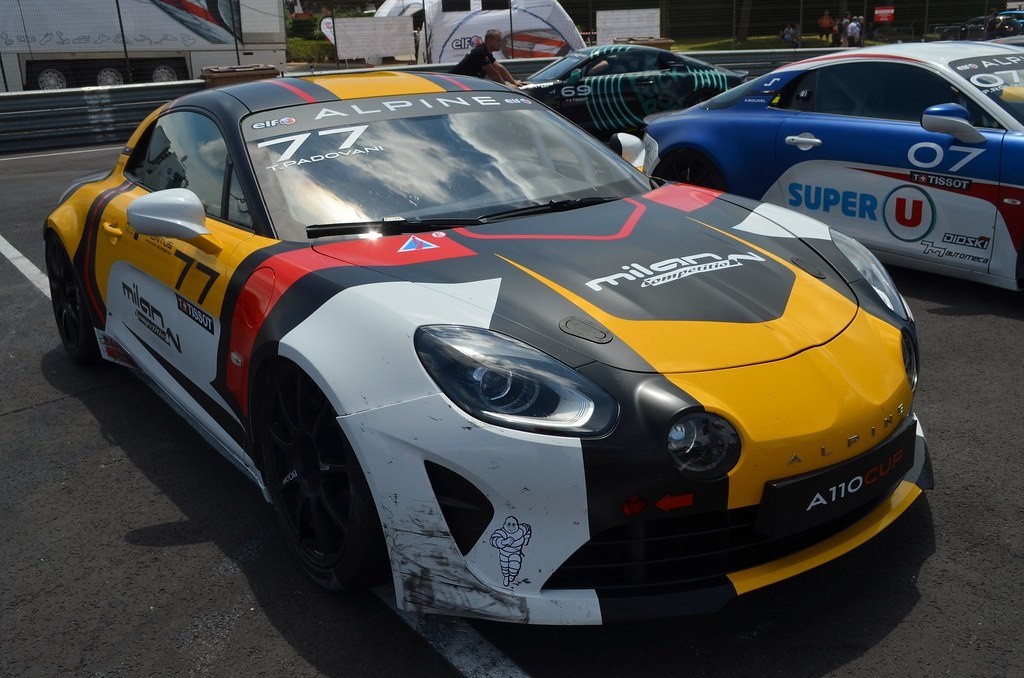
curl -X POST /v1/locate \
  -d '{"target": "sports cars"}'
[500,43,749,137]
[642,39,1024,294]
[43,67,929,629]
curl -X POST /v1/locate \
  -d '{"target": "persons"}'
[449,30,525,90]
[779,2,1008,48]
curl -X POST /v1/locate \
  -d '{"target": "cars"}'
[959,10,1024,47]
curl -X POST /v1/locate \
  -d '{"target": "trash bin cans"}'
[199,64,280,89]
[613,37,675,50]
